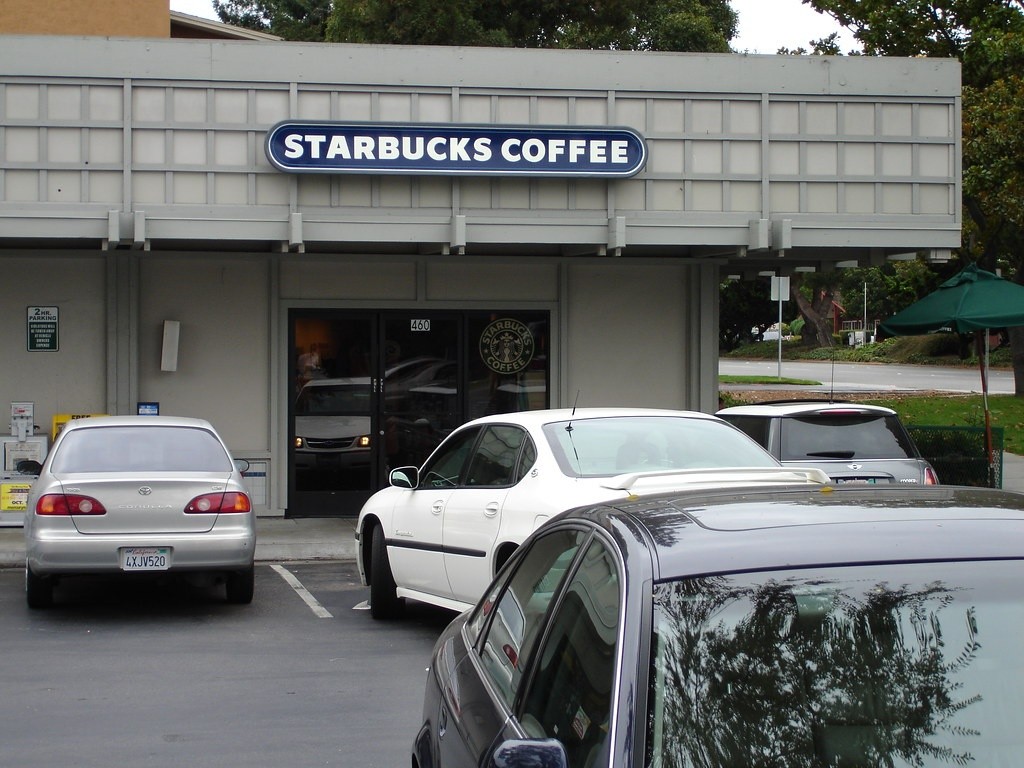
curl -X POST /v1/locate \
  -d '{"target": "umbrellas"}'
[886,259,1024,489]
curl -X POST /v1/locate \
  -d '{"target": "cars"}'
[17,414,256,610]
[293,376,371,488]
[354,407,832,622]
[409,481,1024,768]
[715,398,942,487]
[380,355,488,427]
